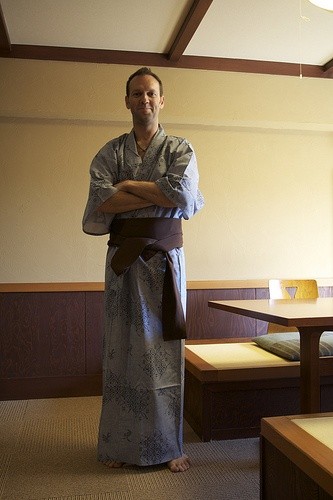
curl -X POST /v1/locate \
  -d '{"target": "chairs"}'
[268,278,318,334]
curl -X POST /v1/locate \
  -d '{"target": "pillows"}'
[252,333,333,361]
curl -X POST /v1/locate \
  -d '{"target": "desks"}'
[208,298,333,415]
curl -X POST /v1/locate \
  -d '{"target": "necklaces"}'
[134,129,158,153]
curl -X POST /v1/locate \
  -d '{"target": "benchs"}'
[182,339,333,443]
[255,414,331,500]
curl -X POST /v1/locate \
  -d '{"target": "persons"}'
[83,66,205,471]
